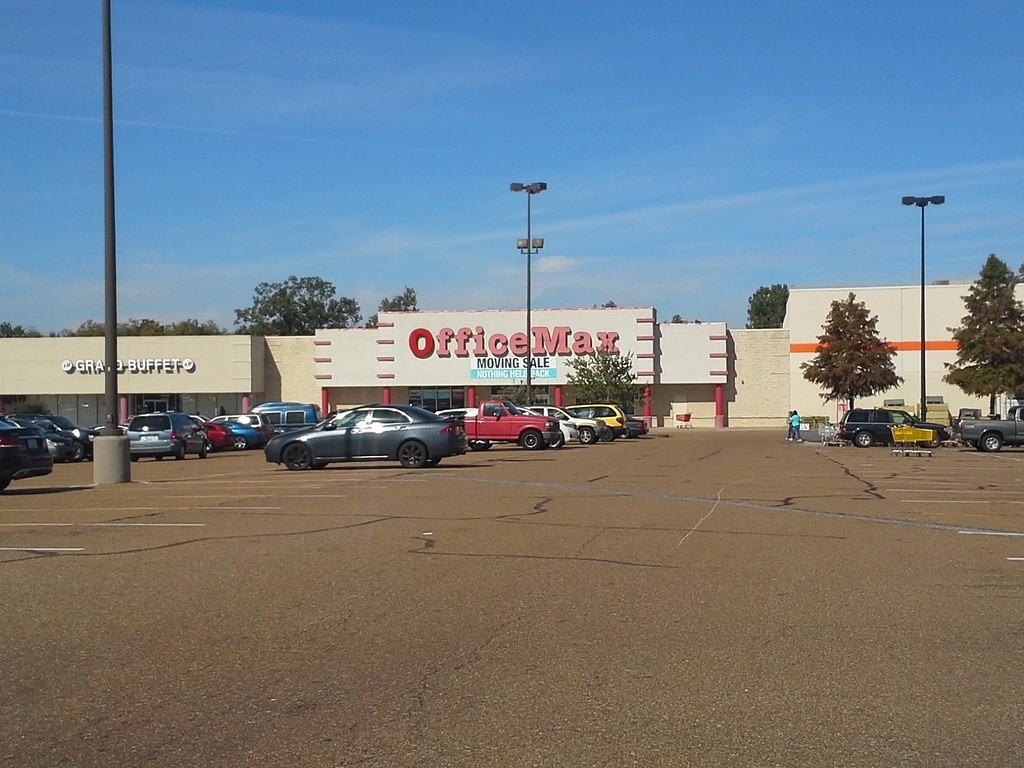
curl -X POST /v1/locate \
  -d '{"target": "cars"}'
[189,412,275,455]
[526,402,648,450]
[0,407,129,492]
[264,402,468,470]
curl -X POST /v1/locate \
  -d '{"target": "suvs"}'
[838,406,950,449]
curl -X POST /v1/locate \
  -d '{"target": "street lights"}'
[510,180,548,405]
[901,194,946,422]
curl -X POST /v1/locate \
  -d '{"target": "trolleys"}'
[888,422,934,457]
[676,411,693,429]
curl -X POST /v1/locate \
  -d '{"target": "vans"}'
[126,412,210,459]
[249,402,317,435]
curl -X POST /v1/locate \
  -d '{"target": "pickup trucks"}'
[459,400,559,453]
[960,405,1024,453]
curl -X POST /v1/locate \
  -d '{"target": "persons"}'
[143,405,151,414]
[785,410,793,440]
[128,410,135,423]
[137,404,145,414]
[220,406,226,416]
[788,410,802,442]
